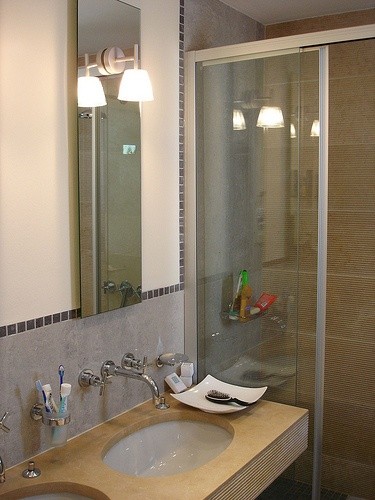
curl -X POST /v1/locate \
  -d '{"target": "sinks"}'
[0,482,112,500]
[101,411,235,477]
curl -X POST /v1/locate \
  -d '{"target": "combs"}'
[205,390,258,406]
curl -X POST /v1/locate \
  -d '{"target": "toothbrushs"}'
[230,272,243,312]
[58,364,64,401]
[36,380,52,425]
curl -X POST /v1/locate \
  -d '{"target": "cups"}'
[40,402,70,445]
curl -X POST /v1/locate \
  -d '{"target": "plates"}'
[170,374,267,414]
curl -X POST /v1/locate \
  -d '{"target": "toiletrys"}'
[41,384,59,418]
[241,285,252,318]
[255,292,276,315]
[228,269,260,320]
[51,383,71,441]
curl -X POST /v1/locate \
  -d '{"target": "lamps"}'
[77,48,107,107]
[231,89,319,138]
[104,44,154,102]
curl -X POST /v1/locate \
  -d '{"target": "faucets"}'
[0,411,10,483]
[100,359,160,399]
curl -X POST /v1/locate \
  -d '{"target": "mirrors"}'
[78,0,142,318]
